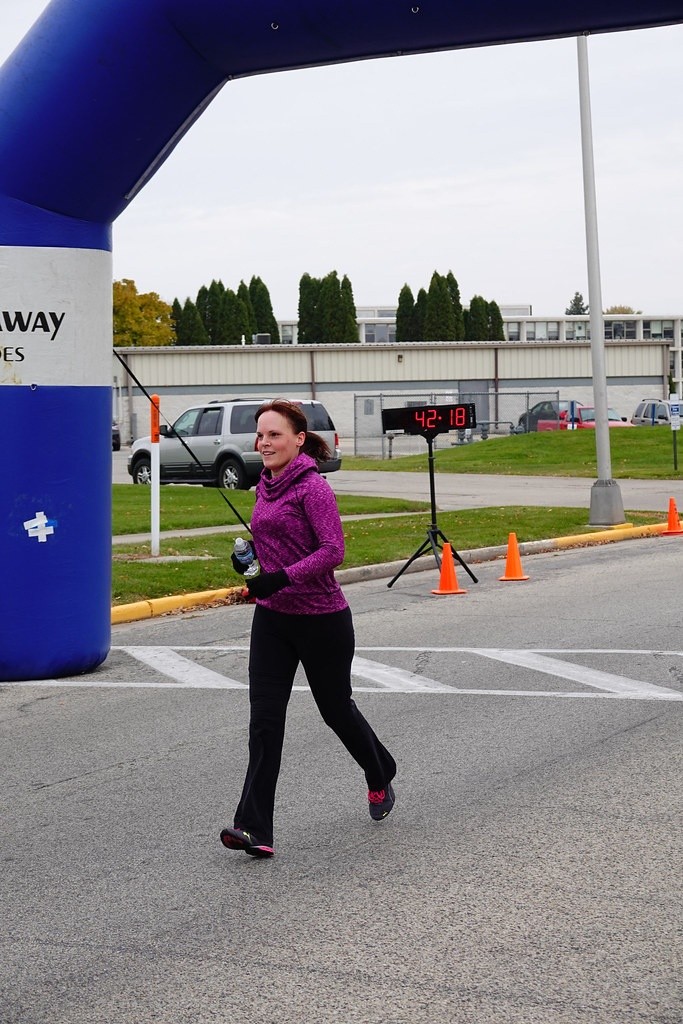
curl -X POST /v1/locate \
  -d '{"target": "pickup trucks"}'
[537,405,635,432]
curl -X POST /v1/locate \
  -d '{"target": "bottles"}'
[233,537,259,576]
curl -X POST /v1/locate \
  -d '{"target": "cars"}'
[112,417,121,452]
[518,399,585,430]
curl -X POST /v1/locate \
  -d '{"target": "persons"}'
[218,394,398,860]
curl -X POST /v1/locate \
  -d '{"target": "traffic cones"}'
[498,532,531,582]
[662,497,682,533]
[432,543,467,595]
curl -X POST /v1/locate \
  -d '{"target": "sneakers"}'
[366,781,396,821]
[219,824,275,858]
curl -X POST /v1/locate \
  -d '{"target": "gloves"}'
[230,538,259,575]
[243,568,292,602]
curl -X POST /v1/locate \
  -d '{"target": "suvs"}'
[630,398,683,426]
[127,396,342,494]
